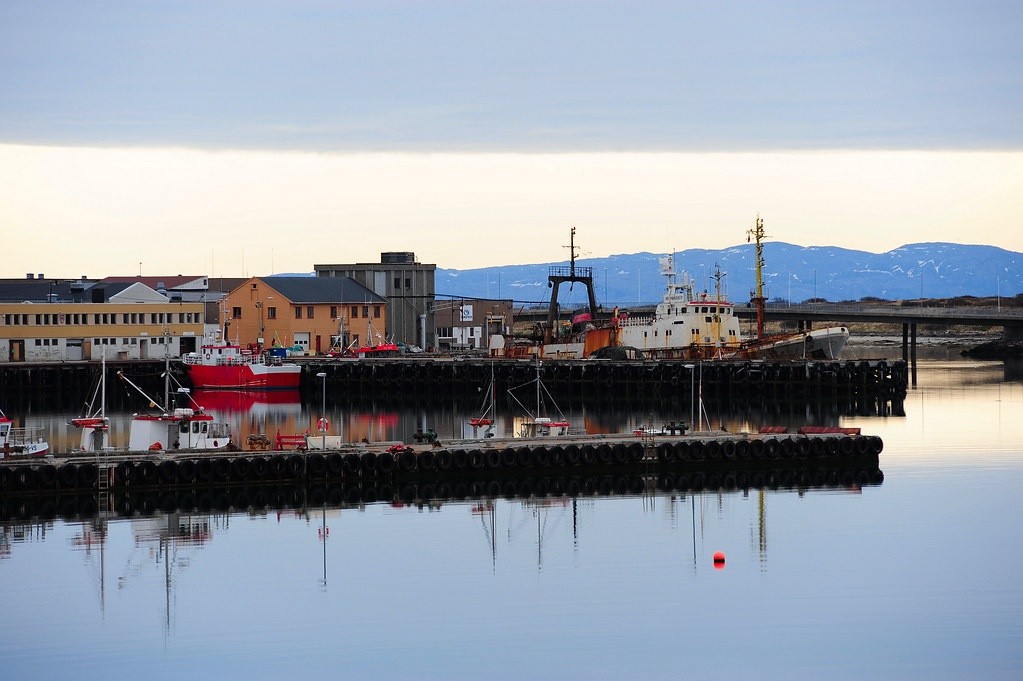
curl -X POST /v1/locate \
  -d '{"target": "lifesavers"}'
[317,418,329,432]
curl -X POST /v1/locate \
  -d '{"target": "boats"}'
[65,328,234,450]
[0,409,50,457]
[179,294,303,391]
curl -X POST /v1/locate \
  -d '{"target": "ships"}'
[479,227,850,361]
